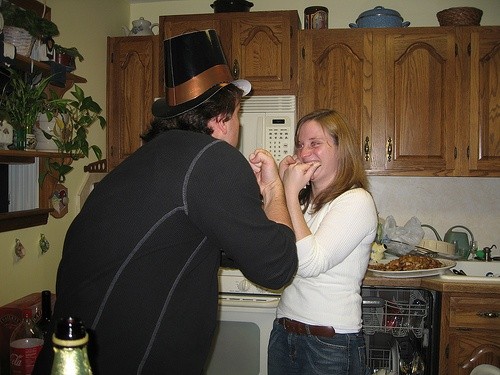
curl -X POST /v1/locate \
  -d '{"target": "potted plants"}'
[36,87,103,187]
[56,41,84,77]
[0,0,56,56]
[0,67,58,151]
[0,89,17,150]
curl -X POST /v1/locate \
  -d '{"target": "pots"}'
[210,0,254,13]
[348,6,411,28]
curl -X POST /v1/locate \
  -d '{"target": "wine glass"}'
[470,241,478,262]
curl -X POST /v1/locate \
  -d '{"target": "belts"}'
[279,317,335,337]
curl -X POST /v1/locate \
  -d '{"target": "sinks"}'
[449,261,500,278]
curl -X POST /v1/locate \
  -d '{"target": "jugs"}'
[420,224,474,261]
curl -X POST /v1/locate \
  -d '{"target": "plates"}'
[367,258,457,278]
[388,340,400,375]
[434,251,462,260]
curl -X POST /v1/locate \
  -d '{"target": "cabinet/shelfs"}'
[472,25,500,170]
[302,27,457,174]
[0,0,88,233]
[160,9,299,88]
[439,283,500,375]
[107,32,163,169]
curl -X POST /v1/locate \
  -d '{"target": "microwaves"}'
[235,94,297,167]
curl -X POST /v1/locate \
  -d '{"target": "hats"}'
[152,28,252,120]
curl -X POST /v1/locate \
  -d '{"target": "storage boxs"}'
[0,290,55,375]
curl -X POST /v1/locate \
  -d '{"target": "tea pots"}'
[120,16,159,36]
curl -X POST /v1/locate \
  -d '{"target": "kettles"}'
[368,242,388,261]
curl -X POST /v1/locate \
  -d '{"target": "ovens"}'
[204,293,280,375]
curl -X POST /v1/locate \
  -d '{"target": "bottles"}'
[9,309,44,375]
[50,315,93,375]
[33,291,53,338]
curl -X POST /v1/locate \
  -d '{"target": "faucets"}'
[483,243,497,262]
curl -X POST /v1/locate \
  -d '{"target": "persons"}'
[267,109,378,375]
[30,28,298,375]
